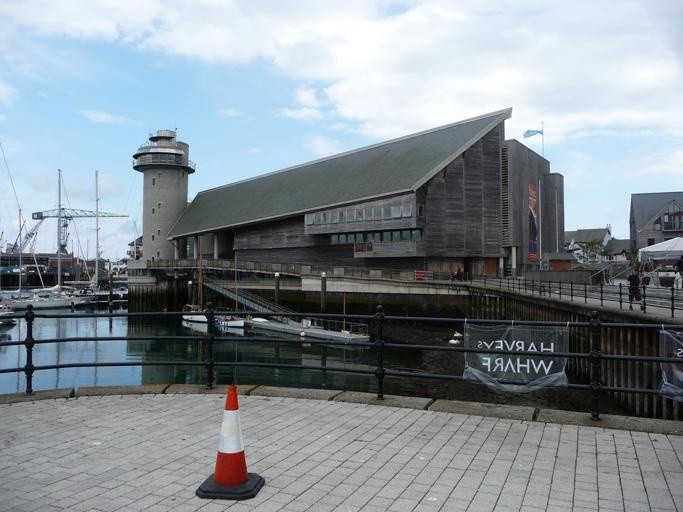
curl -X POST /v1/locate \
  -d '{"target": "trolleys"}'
[447,274,457,281]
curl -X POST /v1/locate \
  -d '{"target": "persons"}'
[529,184,539,261]
[625,268,646,311]
[456,264,464,281]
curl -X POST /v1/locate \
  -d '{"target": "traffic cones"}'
[192,381,266,503]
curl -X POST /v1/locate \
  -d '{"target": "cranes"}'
[31,207,132,254]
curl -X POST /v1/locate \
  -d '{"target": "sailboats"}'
[180,233,246,328]
[6,166,86,312]
[180,320,245,383]
[82,168,128,303]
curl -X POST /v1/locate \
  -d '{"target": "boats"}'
[0,304,16,323]
